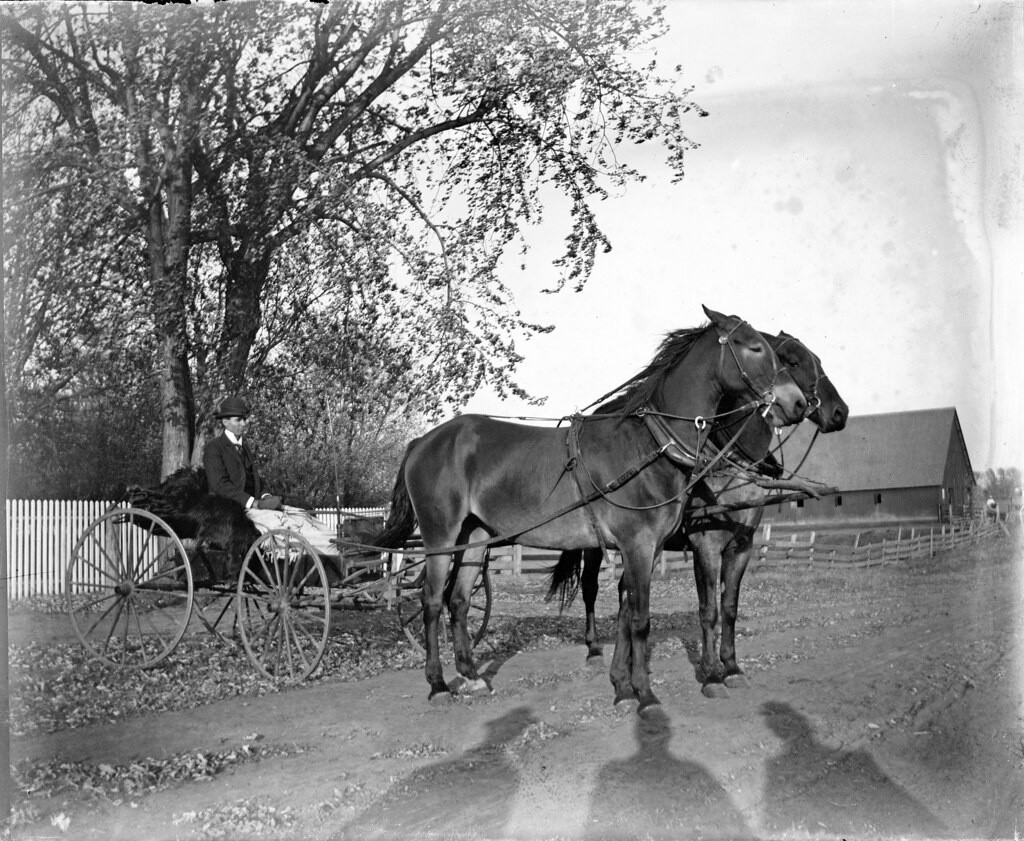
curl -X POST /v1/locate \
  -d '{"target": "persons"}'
[199,396,287,513]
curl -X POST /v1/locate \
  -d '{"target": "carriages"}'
[63,298,852,723]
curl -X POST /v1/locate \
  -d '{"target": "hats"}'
[215,396,249,418]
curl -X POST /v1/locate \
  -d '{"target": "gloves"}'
[257,496,283,511]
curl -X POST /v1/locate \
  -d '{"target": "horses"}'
[357,305,850,721]
